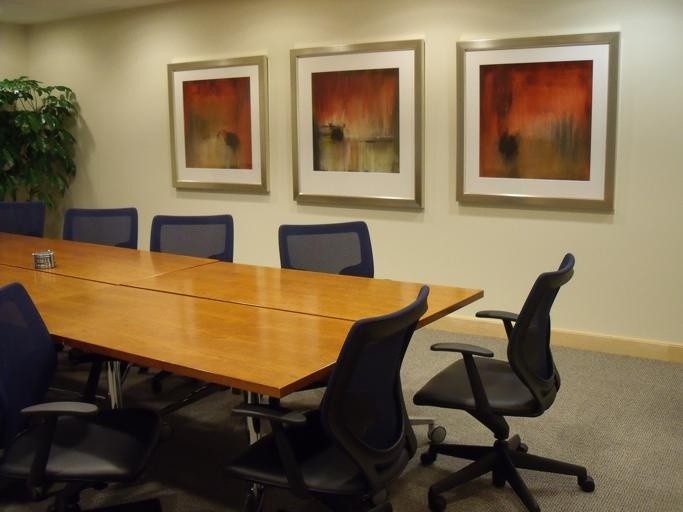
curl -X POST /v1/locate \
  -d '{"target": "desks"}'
[1,233,484,496]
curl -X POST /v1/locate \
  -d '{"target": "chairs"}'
[3,198,46,239]
[413,255,595,512]
[278,223,375,280]
[64,208,136,249]
[1,282,147,510]
[149,215,233,263]
[230,279,432,511]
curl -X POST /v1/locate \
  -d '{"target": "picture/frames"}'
[162,55,269,194]
[288,39,423,213]
[454,31,621,216]
